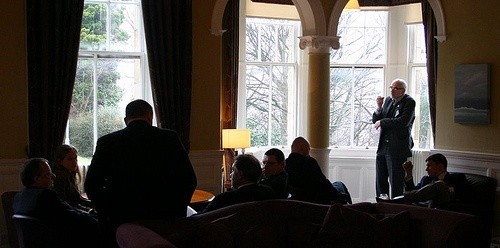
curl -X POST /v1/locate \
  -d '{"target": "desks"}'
[77,189,216,214]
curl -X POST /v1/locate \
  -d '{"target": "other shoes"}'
[376,197,391,202]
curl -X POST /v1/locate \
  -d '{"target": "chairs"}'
[0,172,497,248]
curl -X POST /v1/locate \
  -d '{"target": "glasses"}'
[389,86,404,91]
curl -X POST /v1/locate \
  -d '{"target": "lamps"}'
[221,129,251,157]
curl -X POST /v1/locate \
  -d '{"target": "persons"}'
[372,80,416,198]
[262,148,289,198]
[51,145,92,206]
[84,99,198,248]
[376,153,463,209]
[284,137,352,205]
[205,154,275,211]
[12,158,98,247]
